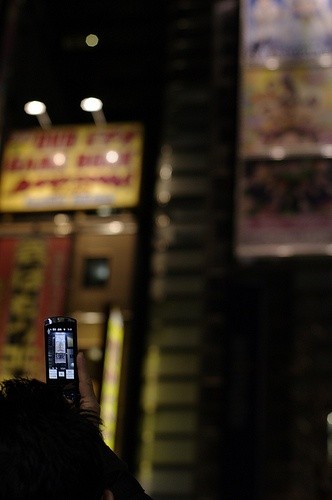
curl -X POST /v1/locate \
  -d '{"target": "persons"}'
[0,352,152,500]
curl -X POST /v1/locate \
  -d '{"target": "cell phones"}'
[44,316,78,405]
